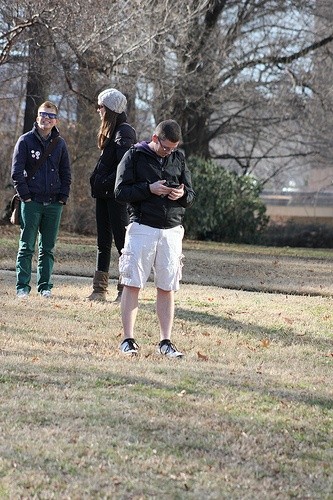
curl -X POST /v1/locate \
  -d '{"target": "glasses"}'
[98,105,103,109]
[157,136,178,152]
[39,112,57,119]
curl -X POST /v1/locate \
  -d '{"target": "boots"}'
[114,277,124,303]
[86,271,110,301]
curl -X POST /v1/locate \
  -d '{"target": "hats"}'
[98,88,127,114]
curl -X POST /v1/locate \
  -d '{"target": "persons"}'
[10,195,21,225]
[12,101,72,296]
[90,88,138,302]
[114,120,196,359]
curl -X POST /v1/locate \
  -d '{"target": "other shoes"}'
[17,292,29,297]
[42,289,52,297]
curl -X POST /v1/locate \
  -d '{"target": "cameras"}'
[164,181,180,190]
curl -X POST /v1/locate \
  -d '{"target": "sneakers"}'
[159,339,183,358]
[119,339,138,356]
